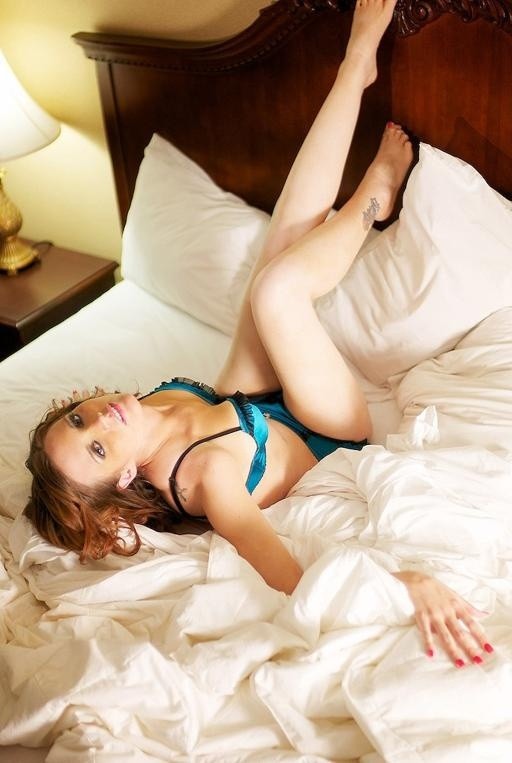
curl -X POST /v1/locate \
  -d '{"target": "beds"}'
[0,0,512,763]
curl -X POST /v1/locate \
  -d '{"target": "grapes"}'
[1,237,120,361]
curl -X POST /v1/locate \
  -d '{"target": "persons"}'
[26,0,496,669]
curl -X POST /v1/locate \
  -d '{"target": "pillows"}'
[313,143,512,388]
[114,124,272,337]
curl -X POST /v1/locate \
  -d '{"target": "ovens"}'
[0,0,512,763]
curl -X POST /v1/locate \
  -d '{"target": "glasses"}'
[0,47,66,274]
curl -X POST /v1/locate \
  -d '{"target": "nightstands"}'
[1,237,120,361]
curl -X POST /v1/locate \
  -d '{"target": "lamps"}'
[0,47,66,274]
